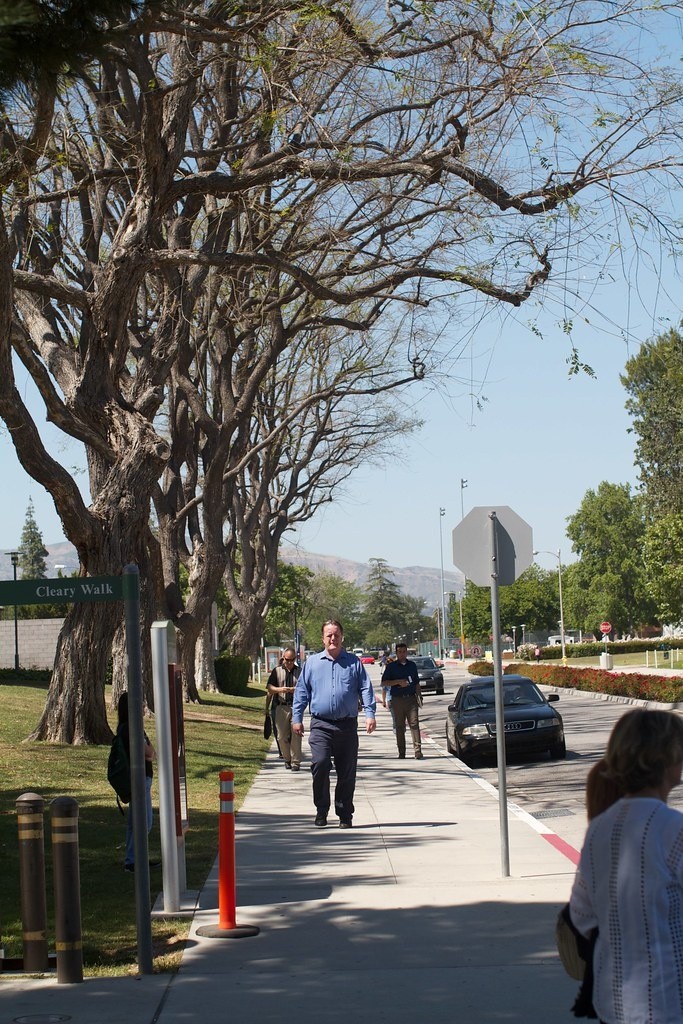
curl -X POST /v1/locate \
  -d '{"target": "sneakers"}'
[285,761,291,769]
[339,819,352,828]
[291,765,300,770]
[315,808,328,826]
[399,753,405,758]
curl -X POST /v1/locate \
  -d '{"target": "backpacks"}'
[106,735,132,815]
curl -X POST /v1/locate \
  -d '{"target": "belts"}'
[314,716,348,722]
[396,694,413,698]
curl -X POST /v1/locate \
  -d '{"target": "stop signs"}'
[600,622,614,635]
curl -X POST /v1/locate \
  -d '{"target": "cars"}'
[353,648,365,656]
[406,656,444,694]
[304,650,320,658]
[358,653,375,665]
[445,674,569,767]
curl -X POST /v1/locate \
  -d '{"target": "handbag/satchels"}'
[264,714,272,739]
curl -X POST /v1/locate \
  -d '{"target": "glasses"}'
[285,658,295,661]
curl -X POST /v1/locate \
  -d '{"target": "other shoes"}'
[415,752,423,759]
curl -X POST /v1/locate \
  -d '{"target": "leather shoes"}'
[124,861,161,873]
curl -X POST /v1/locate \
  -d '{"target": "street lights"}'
[520,624,527,645]
[394,634,407,644]
[438,505,446,658]
[460,479,469,520]
[532,551,569,668]
[444,592,465,662]
[413,628,425,658]
[4,551,26,669]
[511,626,517,659]
[291,600,301,667]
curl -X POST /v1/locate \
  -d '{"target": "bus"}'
[567,629,594,643]
[548,636,576,647]
[368,648,388,655]
[407,648,417,656]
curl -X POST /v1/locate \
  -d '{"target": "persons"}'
[441,647,443,660]
[458,648,462,661]
[265,647,303,772]
[293,619,376,829]
[380,642,424,760]
[445,648,448,658]
[570,708,683,1024]
[116,691,157,874]
[535,645,540,664]
